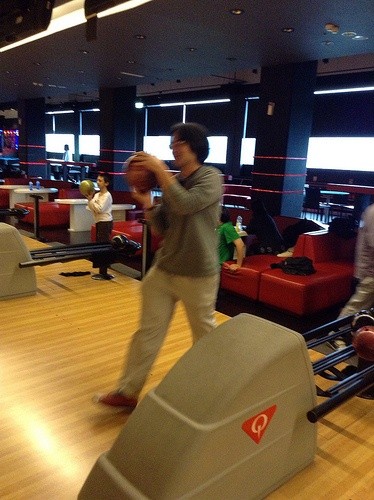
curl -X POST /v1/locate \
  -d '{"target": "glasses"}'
[170,139,185,149]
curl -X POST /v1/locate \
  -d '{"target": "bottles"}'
[236,214,243,230]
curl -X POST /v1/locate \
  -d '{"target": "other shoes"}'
[326,331,347,351]
[91,391,138,410]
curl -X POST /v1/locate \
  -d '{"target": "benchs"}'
[218,174,358,316]
[0,178,157,258]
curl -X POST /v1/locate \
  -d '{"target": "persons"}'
[328,205,374,348]
[85,173,112,280]
[242,201,285,255]
[215,206,244,271]
[62,145,72,181]
[97,123,223,407]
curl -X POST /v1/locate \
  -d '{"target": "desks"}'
[0,184,48,210]
[307,179,374,226]
[13,188,58,237]
[48,158,97,179]
[54,198,136,232]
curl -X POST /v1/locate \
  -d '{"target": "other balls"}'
[111,234,129,250]
[120,155,161,193]
[351,306,374,361]
[79,180,95,196]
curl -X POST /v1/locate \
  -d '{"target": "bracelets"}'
[142,205,156,212]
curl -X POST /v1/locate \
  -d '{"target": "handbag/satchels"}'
[271,256,314,276]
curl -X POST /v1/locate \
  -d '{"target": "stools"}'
[312,201,355,222]
